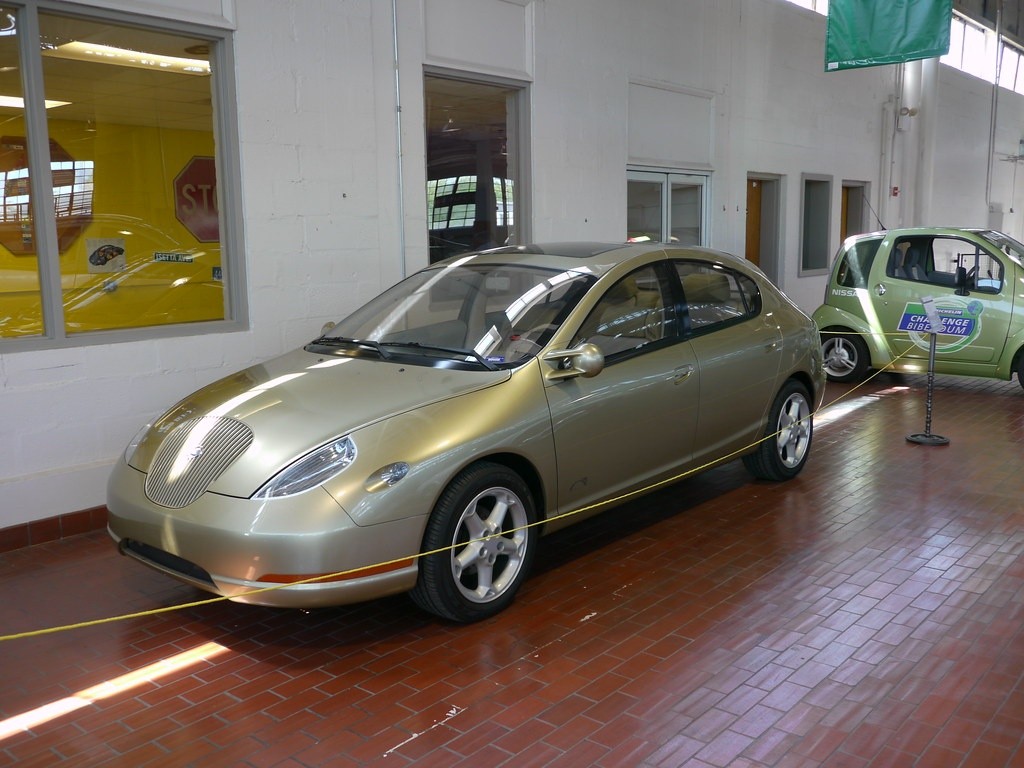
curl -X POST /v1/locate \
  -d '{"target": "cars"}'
[811,227,1024,390]
[105,242,827,624]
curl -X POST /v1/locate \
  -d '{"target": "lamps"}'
[86,118,96,132]
[500,143,507,155]
[442,118,460,132]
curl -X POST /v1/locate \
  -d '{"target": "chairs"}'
[497,308,544,354]
[895,249,907,277]
[903,246,930,282]
[586,307,650,357]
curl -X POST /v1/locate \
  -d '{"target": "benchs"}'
[585,275,738,340]
